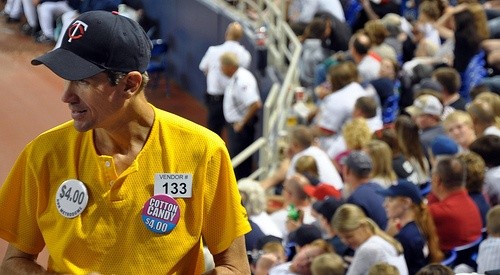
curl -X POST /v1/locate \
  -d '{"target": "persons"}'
[199,21,252,137]
[219,53,261,180]
[0,10,252,275]
[0,0,122,53]
[204,0,500,275]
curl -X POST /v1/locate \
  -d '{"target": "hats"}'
[315,197,342,219]
[288,225,321,244]
[377,179,423,206]
[30,10,151,81]
[340,153,371,177]
[405,95,443,117]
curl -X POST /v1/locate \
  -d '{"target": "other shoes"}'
[0,10,52,44]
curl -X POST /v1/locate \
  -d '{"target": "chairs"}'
[382,51,487,272]
[139,17,173,100]
